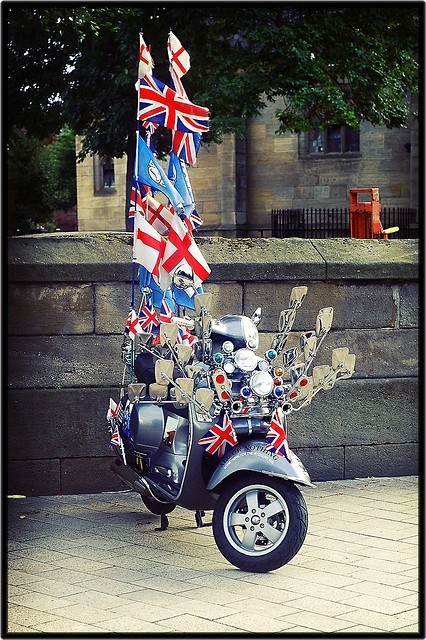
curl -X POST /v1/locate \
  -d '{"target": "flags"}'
[137,32,153,78]
[108,424,121,451]
[123,74,212,349]
[106,397,119,422]
[166,29,193,79]
[264,409,292,464]
[195,412,239,459]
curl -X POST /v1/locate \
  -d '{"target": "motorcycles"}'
[106,285,358,574]
